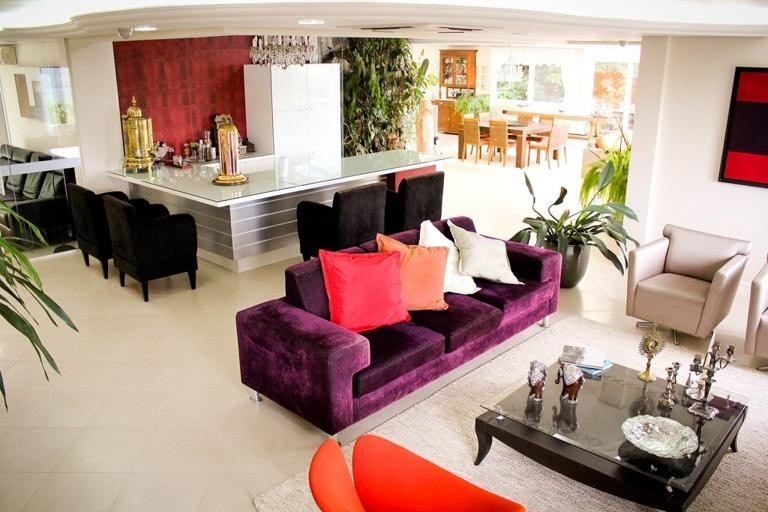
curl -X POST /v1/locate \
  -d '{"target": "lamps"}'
[246,35,315,70]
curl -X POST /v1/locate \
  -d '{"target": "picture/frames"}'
[716,66,768,188]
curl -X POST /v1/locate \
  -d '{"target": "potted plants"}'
[505,159,643,288]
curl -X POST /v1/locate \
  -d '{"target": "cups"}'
[172,155,182,166]
[183,139,217,162]
[238,146,247,156]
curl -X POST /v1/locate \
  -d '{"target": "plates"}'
[618,439,695,478]
[621,414,699,458]
[685,387,714,402]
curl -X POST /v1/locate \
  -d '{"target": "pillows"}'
[417,219,483,296]
[317,245,412,335]
[375,231,451,314]
[446,218,527,286]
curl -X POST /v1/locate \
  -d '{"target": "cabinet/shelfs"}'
[237,157,274,177]
[430,49,478,135]
[236,62,344,178]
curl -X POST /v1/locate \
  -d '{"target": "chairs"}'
[66,181,151,281]
[385,169,447,235]
[458,108,631,184]
[743,251,768,373]
[624,223,755,346]
[295,179,387,264]
[306,431,525,511]
[102,193,200,304]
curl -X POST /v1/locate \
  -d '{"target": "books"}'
[560,345,614,378]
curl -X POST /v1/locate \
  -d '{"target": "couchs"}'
[0,142,81,252]
[234,215,564,449]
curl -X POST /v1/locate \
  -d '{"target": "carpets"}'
[248,310,768,512]
